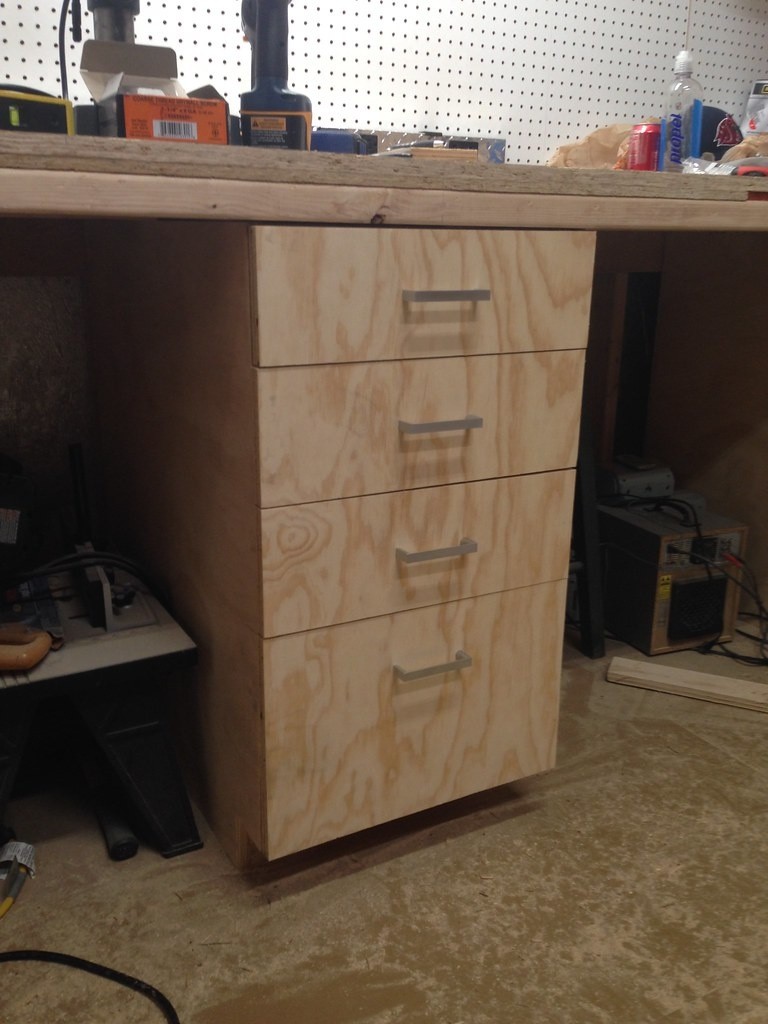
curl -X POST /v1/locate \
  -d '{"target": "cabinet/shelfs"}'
[83,217,595,868]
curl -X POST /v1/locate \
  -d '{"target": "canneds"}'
[627,123,661,172]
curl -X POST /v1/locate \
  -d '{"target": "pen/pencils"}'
[391,140,445,148]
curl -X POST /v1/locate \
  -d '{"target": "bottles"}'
[658,51,704,174]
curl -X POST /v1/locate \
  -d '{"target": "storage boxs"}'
[79,39,232,145]
